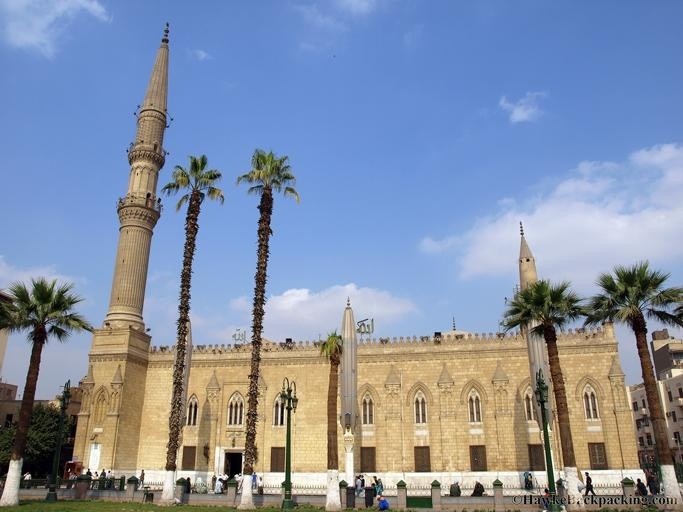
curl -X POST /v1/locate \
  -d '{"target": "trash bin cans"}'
[346,487,355,508]
[148,493,153,502]
[364,487,374,508]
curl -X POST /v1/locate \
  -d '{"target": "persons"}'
[377,496,389,511]
[214,473,228,493]
[524,470,533,489]
[24,472,32,489]
[355,475,364,496]
[186,477,191,504]
[252,472,258,489]
[86,469,112,479]
[372,476,384,498]
[544,488,549,496]
[69,472,78,481]
[258,477,263,496]
[139,469,144,487]
[636,478,648,496]
[56,476,60,489]
[584,472,597,496]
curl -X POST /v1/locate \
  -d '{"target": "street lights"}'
[535,368,561,512]
[45,379,71,502]
[281,377,298,512]
